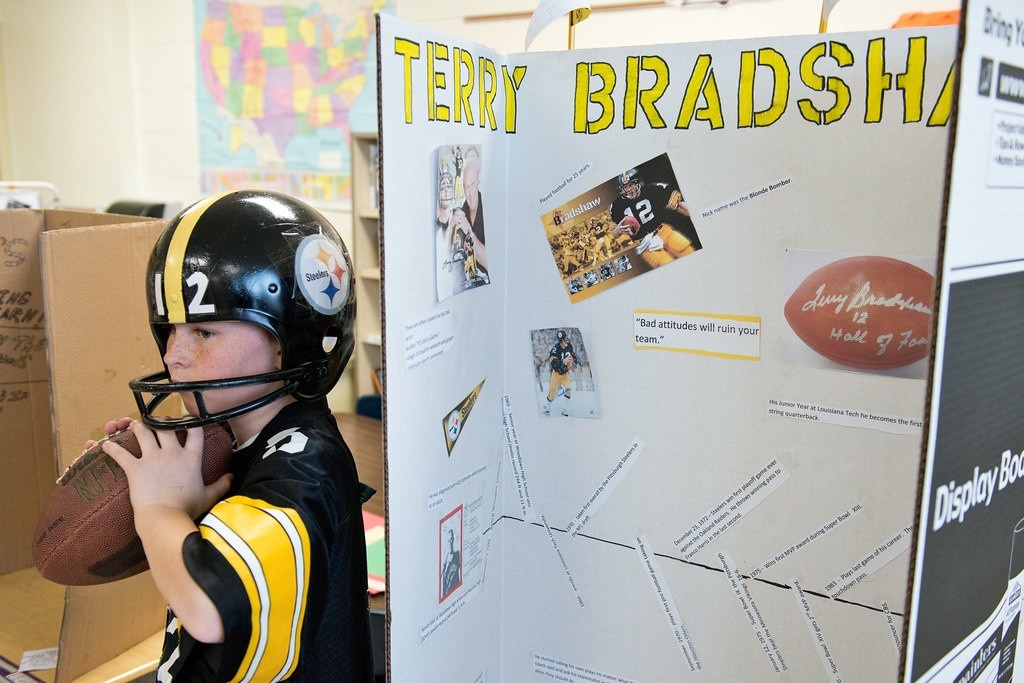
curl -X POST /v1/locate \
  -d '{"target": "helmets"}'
[146,190,357,403]
[439,160,456,187]
[556,331,567,341]
[618,169,645,191]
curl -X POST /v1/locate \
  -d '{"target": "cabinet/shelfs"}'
[349,129,380,417]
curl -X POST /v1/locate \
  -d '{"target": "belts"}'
[652,225,662,237]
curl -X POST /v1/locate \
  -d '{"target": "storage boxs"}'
[0,207,172,683]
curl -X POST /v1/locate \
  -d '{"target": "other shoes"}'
[543,405,550,415]
[562,407,569,416]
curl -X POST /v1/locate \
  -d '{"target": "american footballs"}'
[780,251,943,375]
[566,355,575,371]
[25,413,241,592]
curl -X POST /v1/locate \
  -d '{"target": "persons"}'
[441,527,461,597]
[435,144,491,285]
[541,330,582,417]
[78,190,376,683]
[549,170,691,294]
